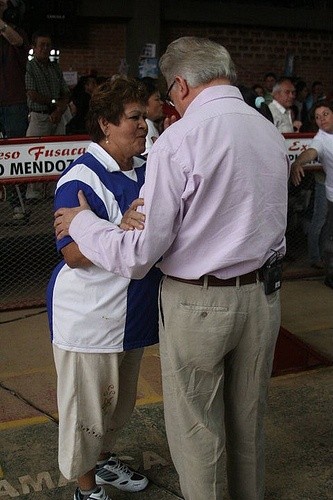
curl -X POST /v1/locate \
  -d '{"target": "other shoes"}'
[13,207,30,219]
[311,262,325,269]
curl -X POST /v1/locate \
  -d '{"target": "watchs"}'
[50,98,57,107]
[0,24,8,32]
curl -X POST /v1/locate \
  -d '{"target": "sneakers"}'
[95,455,149,492]
[74,486,111,500]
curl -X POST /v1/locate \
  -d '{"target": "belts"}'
[165,265,265,287]
[32,110,51,115]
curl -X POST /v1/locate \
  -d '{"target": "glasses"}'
[37,41,51,47]
[163,81,178,106]
[315,87,322,90]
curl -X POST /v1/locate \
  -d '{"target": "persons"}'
[45,77,177,500]
[0,0,332,225]
[53,35,291,500]
[291,96,333,290]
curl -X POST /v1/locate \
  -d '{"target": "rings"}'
[127,205,136,210]
